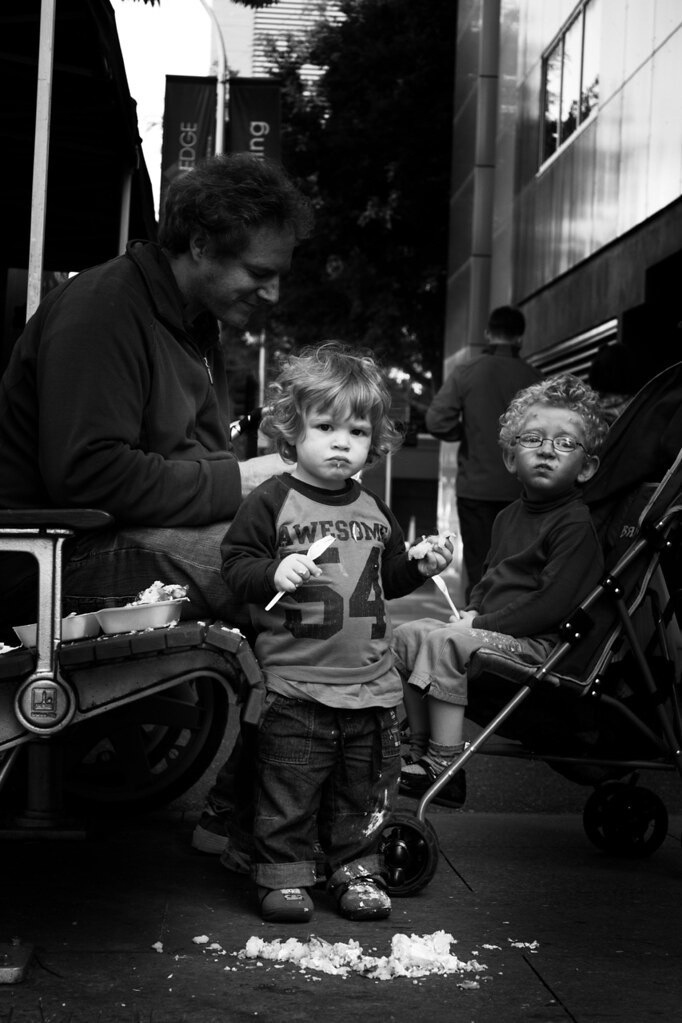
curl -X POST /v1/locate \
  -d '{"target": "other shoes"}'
[398,758,466,808]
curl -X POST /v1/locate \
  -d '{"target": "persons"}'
[0,153,312,613]
[392,376,623,807]
[221,344,454,921]
[425,306,544,606]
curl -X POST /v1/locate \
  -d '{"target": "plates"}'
[94,600,185,635]
[11,611,100,648]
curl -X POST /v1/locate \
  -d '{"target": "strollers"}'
[373,357,682,899]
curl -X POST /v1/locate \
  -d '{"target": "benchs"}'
[0,503,268,842]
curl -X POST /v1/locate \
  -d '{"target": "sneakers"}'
[257,880,314,923]
[340,877,391,918]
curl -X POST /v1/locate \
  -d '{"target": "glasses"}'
[516,432,587,458]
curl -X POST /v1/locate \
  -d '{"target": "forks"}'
[264,536,336,612]
[431,576,461,623]
